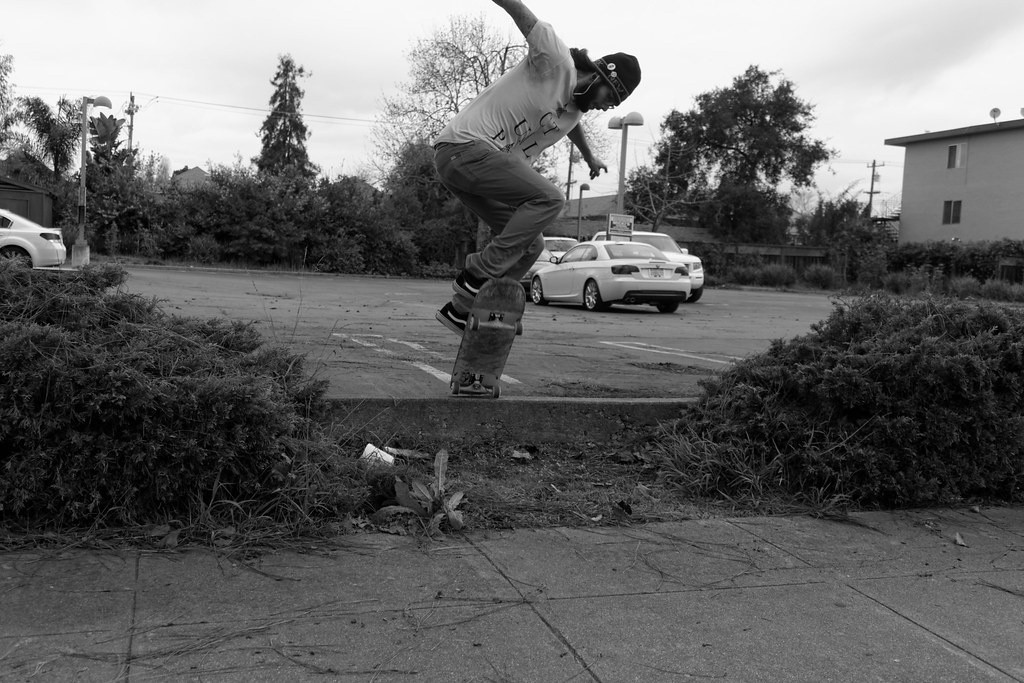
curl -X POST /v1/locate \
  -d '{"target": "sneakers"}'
[435,301,469,337]
[452,269,483,302]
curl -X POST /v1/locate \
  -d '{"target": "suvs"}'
[591,231,704,303]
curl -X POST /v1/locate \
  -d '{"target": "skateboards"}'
[449,274,526,398]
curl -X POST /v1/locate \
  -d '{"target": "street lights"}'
[609,112,643,214]
[71,96,111,268]
[577,184,589,242]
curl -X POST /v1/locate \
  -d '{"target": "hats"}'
[592,52,642,106]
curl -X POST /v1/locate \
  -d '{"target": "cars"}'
[520,237,579,296]
[0,209,66,271]
[529,241,692,313]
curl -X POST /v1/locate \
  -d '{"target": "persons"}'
[432,0,642,339]
